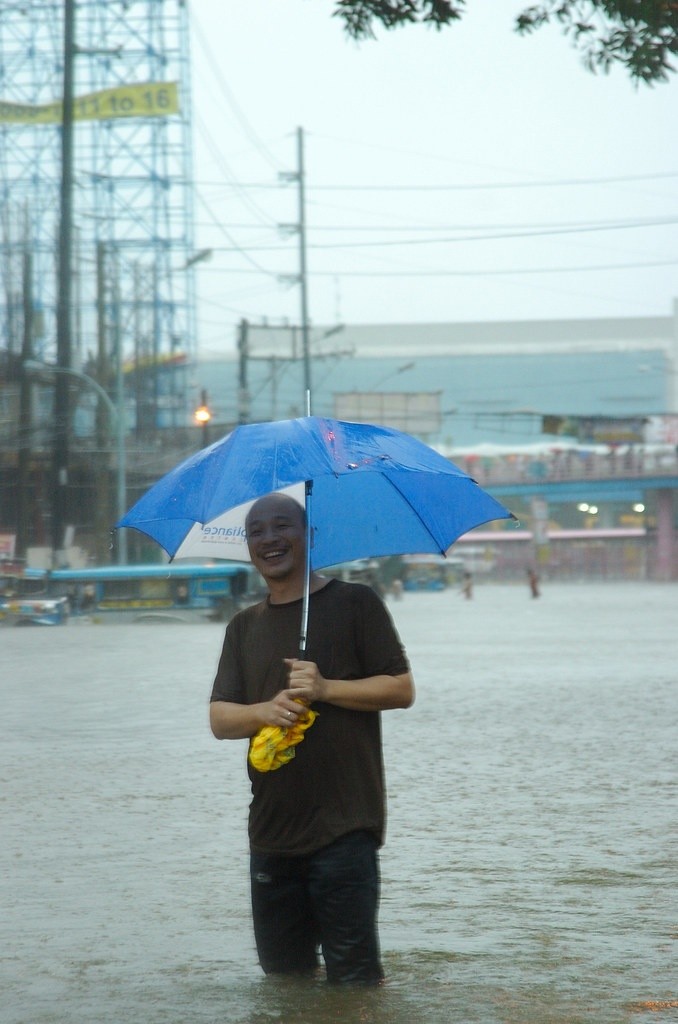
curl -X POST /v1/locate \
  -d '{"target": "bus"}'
[398,559,472,592]
[0,563,252,630]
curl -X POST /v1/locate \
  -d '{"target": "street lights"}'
[24,359,129,568]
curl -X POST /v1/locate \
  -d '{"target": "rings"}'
[288,712,291,716]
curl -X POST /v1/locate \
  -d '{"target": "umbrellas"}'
[111,387,519,660]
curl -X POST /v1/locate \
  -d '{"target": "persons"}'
[463,573,472,598]
[528,570,540,597]
[465,447,645,478]
[390,574,402,602]
[209,493,415,982]
